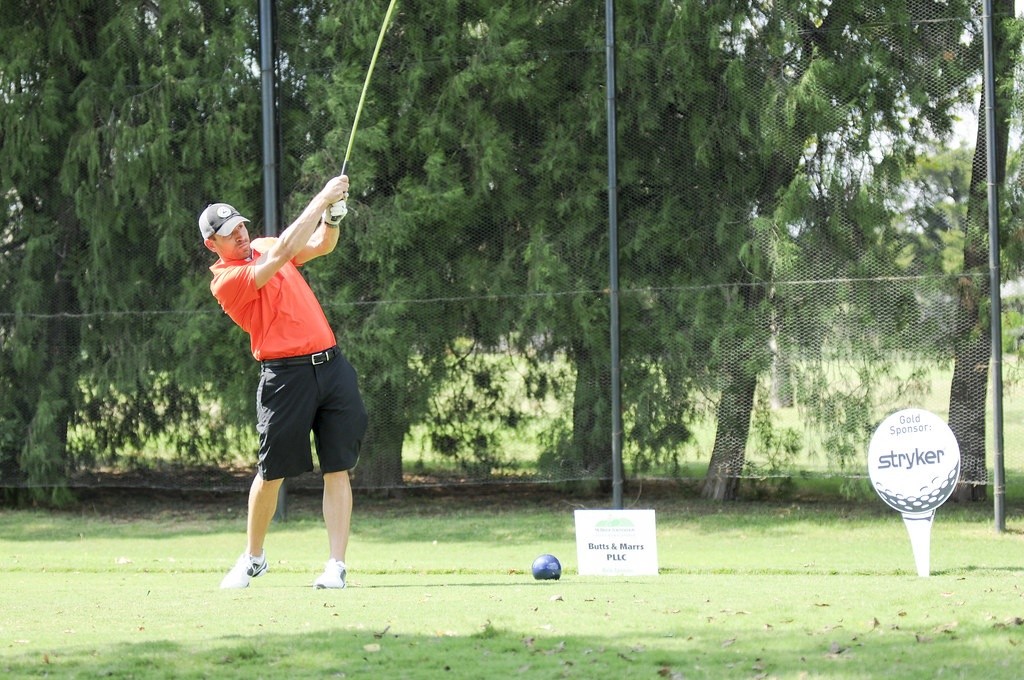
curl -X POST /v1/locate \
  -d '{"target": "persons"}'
[199,173,369,589]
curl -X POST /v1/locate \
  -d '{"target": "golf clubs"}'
[330,0,397,221]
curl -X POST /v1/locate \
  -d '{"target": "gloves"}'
[323,193,349,227]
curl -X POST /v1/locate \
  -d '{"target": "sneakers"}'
[316,560,347,589]
[222,549,268,590]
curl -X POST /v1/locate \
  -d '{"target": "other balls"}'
[531,553,562,581]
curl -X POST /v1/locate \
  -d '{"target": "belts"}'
[261,346,339,368]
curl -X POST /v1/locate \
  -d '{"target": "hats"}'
[199,203,251,239]
